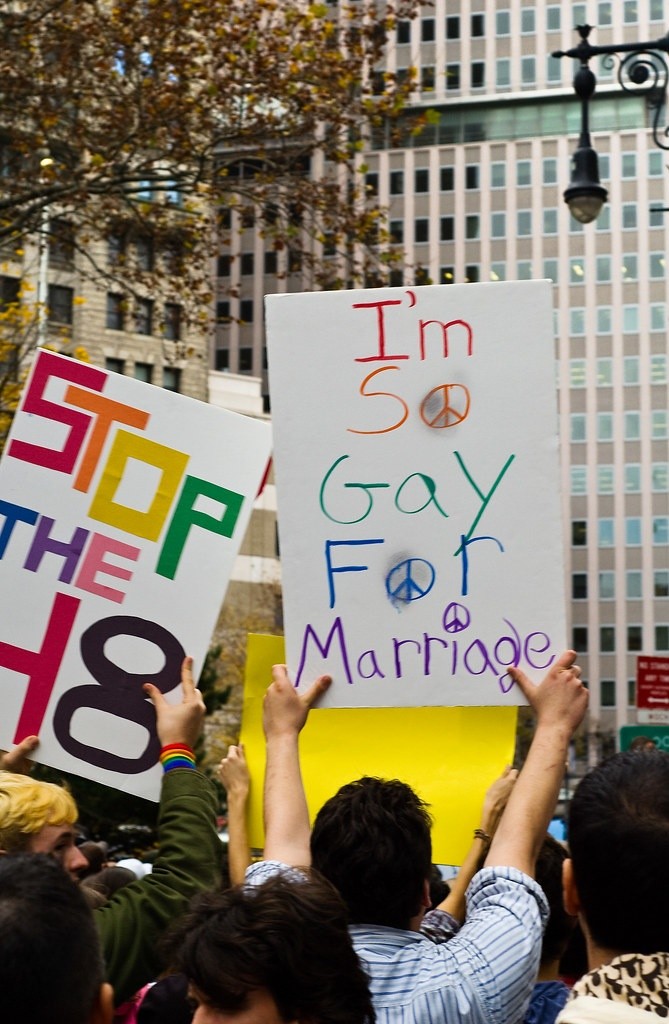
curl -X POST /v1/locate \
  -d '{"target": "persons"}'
[0,657,669,1024]
[245,647,590,1024]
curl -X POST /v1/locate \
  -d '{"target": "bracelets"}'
[473,829,492,843]
